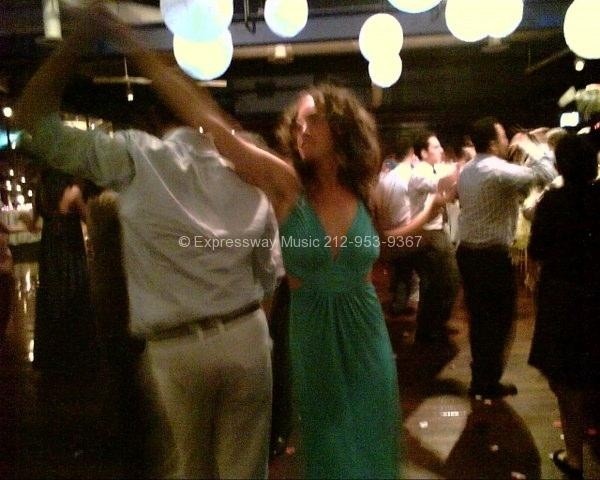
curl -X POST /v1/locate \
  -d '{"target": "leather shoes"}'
[380,301,520,398]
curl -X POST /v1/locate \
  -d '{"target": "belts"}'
[145,299,264,342]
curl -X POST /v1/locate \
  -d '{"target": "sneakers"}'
[552,447,585,478]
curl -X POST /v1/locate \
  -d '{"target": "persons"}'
[375,114,600,406]
[0,163,137,464]
[13,2,282,480]
[57,0,456,480]
[524,132,600,480]
